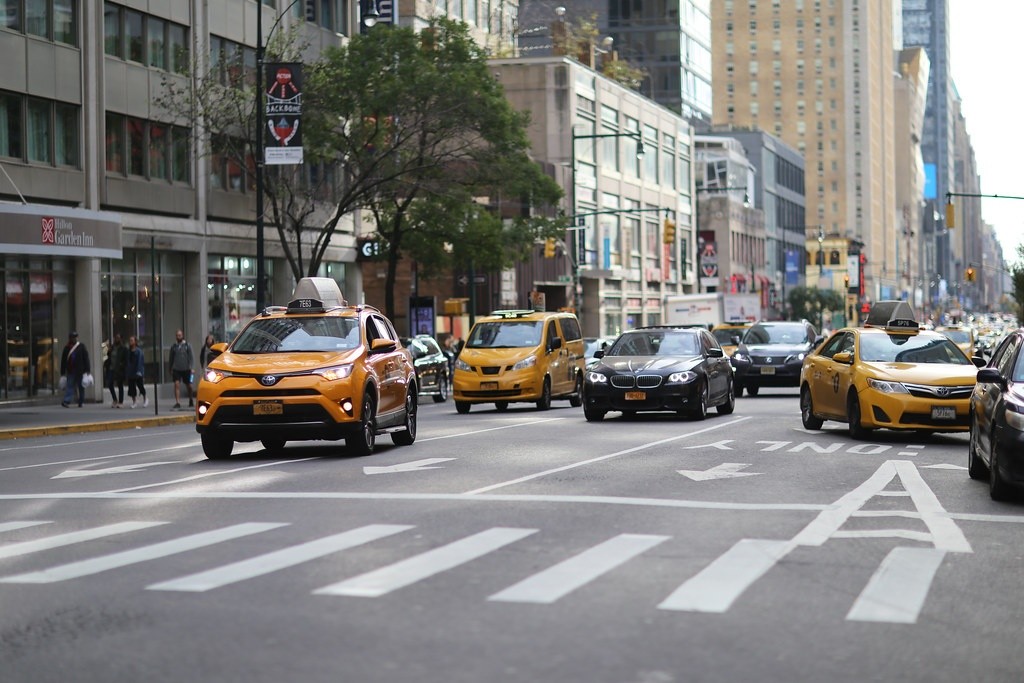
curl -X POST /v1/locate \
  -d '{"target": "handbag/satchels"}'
[57,375,68,392]
[81,372,95,389]
[104,358,110,368]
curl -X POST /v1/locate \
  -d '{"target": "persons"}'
[168,329,196,409]
[106,334,149,409]
[59,331,92,409]
[201,334,219,370]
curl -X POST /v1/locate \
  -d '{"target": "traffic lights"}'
[544,237,556,259]
[663,218,675,244]
[964,269,976,281]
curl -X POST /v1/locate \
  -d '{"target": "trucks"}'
[665,293,762,326]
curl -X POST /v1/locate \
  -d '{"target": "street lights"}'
[572,130,646,314]
[782,226,825,319]
[695,184,750,294]
[254,0,380,312]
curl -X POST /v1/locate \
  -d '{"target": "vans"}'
[453,311,587,413]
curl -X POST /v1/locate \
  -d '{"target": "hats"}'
[69,332,79,338]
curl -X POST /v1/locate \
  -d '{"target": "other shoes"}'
[61,400,70,408]
[173,403,181,409]
[111,400,118,407]
[131,402,138,409]
[142,398,148,408]
[117,403,124,409]
[77,399,83,408]
[189,398,194,407]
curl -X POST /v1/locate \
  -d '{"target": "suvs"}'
[195,277,419,462]
[398,333,450,403]
[966,326,1024,499]
[733,315,819,398]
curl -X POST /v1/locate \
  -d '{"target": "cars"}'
[935,312,1017,363]
[710,326,752,358]
[799,300,979,441]
[584,324,735,421]
[584,337,616,370]
